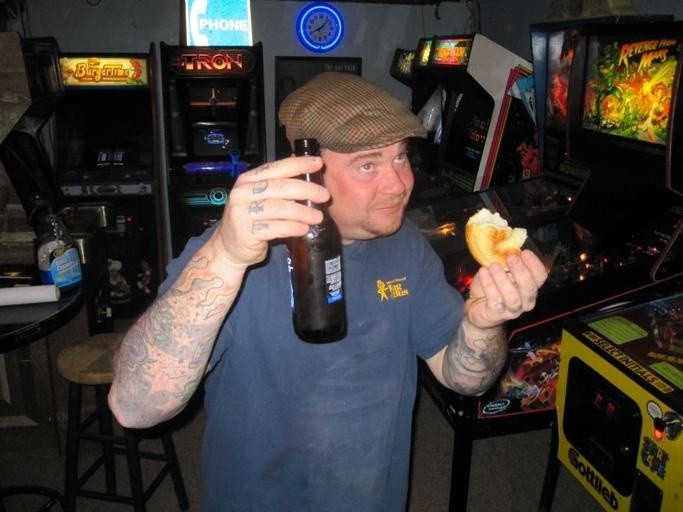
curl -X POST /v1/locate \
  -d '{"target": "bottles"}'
[285,138,347,343]
[35,203,84,292]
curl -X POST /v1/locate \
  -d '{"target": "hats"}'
[278,70,428,153]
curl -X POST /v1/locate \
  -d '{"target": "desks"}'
[0,264,83,512]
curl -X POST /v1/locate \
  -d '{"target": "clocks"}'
[296,2,344,53]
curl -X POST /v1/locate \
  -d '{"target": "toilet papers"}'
[0,285,60,306]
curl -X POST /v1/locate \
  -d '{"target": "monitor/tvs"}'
[459,110,491,170]
[187,118,242,159]
[55,102,149,180]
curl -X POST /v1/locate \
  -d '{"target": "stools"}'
[57,333,190,512]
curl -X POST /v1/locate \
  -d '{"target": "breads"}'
[464,209,528,271]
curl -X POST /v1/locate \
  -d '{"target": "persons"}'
[107,71,549,512]
